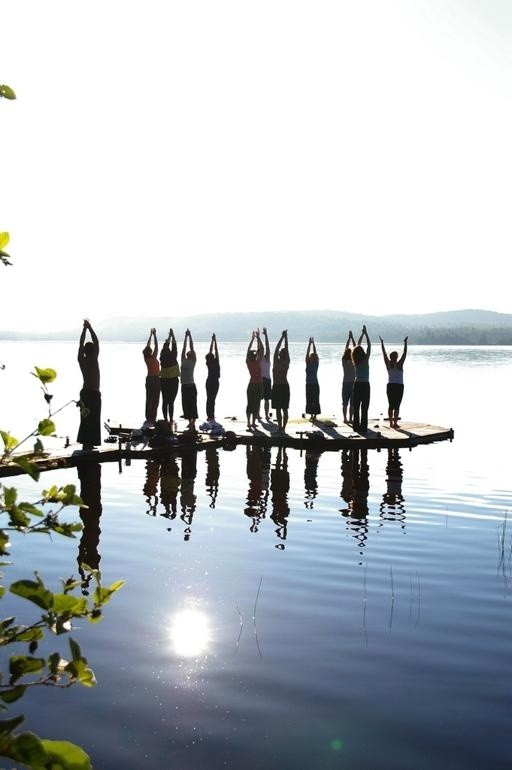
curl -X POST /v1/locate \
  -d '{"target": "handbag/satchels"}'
[130,419,202,446]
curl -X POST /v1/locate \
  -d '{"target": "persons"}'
[352,324,372,433]
[256,327,272,420]
[205,334,221,421]
[379,335,409,427]
[342,330,357,423]
[77,317,101,450]
[246,330,261,427]
[180,330,199,427]
[305,335,321,421]
[161,328,177,420]
[143,328,160,426]
[272,329,290,432]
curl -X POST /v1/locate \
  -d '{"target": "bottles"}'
[378,413,385,429]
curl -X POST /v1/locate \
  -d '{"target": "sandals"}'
[104,436,117,443]
